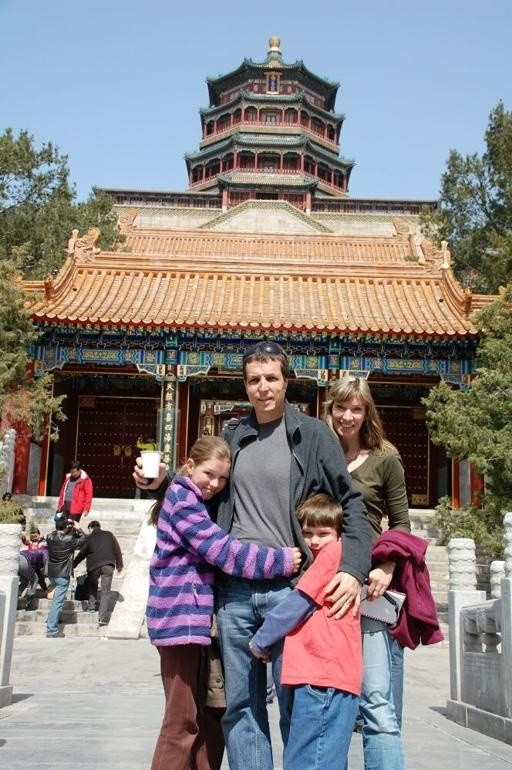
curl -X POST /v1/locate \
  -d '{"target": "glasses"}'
[244,342,286,358]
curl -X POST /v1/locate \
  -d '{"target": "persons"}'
[1,459,124,639]
[132,340,410,770]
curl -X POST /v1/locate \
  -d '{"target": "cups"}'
[141,450,163,479]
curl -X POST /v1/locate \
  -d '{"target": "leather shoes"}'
[25,603,36,611]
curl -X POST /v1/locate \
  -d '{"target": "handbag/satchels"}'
[75,574,89,600]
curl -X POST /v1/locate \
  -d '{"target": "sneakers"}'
[47,631,65,638]
[84,608,95,613]
